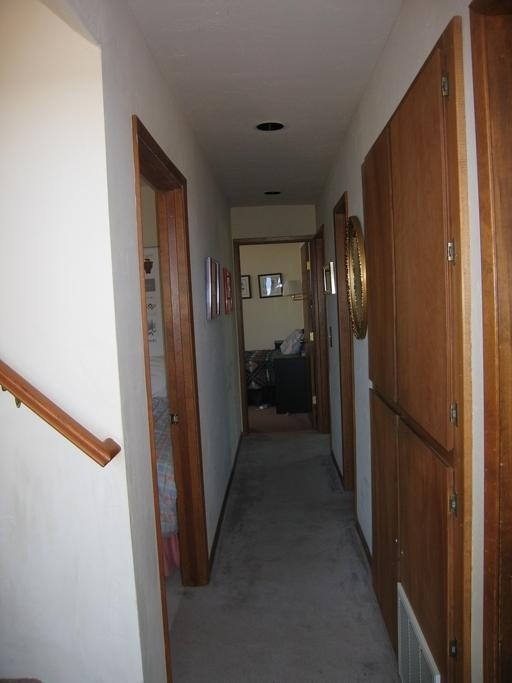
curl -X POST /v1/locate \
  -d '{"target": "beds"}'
[244,328,314,415]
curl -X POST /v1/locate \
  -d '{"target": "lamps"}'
[282,278,304,301]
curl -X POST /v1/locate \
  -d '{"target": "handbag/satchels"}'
[279,329,305,355]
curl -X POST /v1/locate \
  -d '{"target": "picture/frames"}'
[322,261,336,295]
[204,256,234,321]
[344,215,371,341]
[240,272,284,300]
